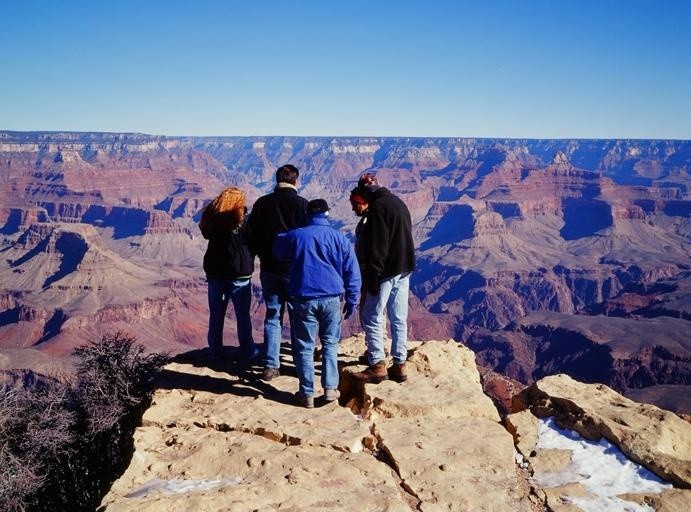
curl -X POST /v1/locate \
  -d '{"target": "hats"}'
[307,199,331,215]
[350,188,367,205]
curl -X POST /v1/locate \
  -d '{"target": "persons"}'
[246,164,318,382]
[348,188,388,365]
[272,199,362,409]
[349,174,415,384]
[198,188,259,361]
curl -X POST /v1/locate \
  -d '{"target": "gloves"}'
[343,303,356,320]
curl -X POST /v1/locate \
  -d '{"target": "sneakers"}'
[324,389,341,401]
[243,348,260,360]
[352,361,388,382]
[263,368,280,381]
[387,364,406,382]
[295,392,315,409]
